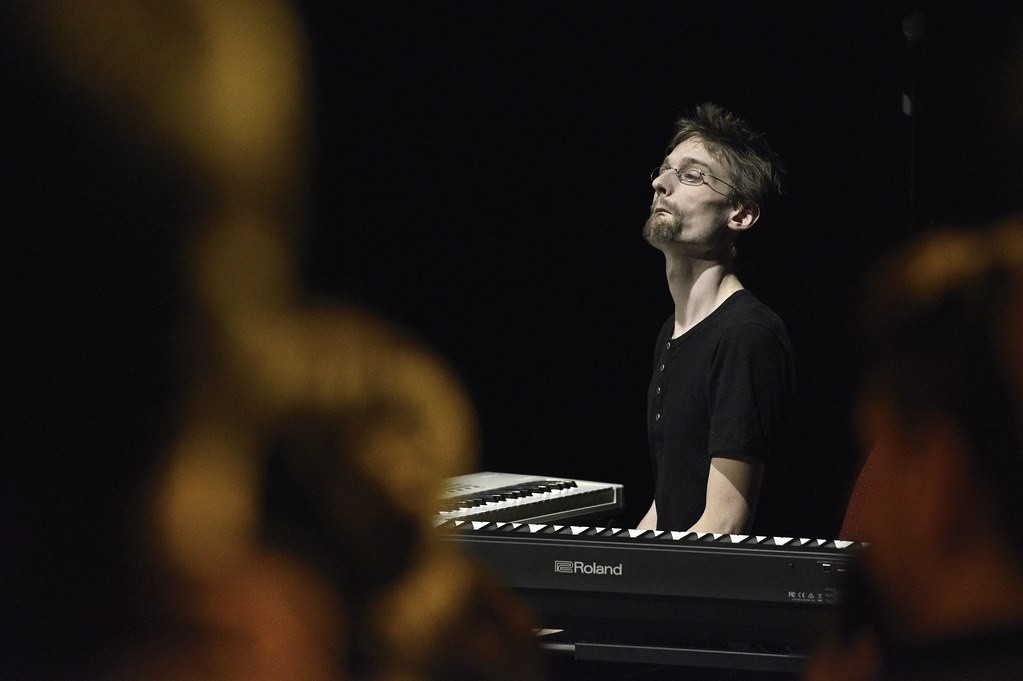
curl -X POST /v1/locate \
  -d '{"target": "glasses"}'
[651,166,757,203]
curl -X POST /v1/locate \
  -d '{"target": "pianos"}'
[420,463,892,678]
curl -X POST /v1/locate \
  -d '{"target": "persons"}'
[635,103,800,535]
